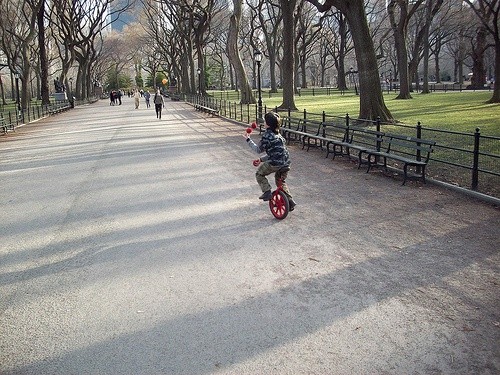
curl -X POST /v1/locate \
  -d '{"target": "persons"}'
[153,90,165,119]
[143,89,151,108]
[242,112,296,211]
[109,88,144,109]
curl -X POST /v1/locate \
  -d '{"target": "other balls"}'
[250,122,257,128]
[246,128,252,133]
[253,160,258,166]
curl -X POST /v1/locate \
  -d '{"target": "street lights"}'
[14,70,21,115]
[252,50,265,123]
[197,68,202,104]
[69,77,73,102]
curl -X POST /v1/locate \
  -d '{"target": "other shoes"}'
[259,190,272,199]
[288,199,296,212]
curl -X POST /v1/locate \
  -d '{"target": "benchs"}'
[47,100,72,117]
[258,115,436,185]
[194,100,220,118]
[0,118,15,135]
